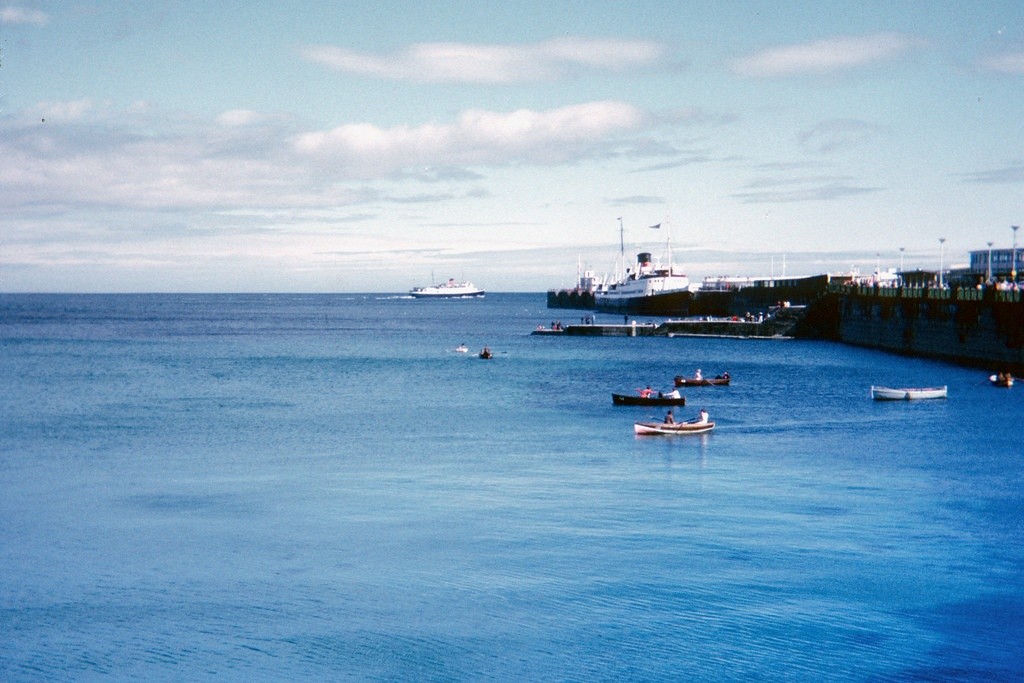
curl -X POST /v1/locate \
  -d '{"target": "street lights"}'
[939,238,946,289]
[987,242,993,283]
[1011,225,1020,280]
[900,248,904,272]
[877,254,879,268]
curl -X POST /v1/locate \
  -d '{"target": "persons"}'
[638,385,657,398]
[662,387,681,399]
[581,312,596,326]
[623,313,628,325]
[663,410,674,426]
[695,409,708,426]
[695,369,703,380]
[997,372,1012,381]
[715,370,729,380]
[551,320,562,331]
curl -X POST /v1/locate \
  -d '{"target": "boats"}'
[871,386,947,399]
[990,373,1014,388]
[479,346,492,359]
[674,376,730,387]
[455,343,468,353]
[611,393,685,407]
[634,422,715,435]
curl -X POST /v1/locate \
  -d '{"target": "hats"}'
[696,369,701,371]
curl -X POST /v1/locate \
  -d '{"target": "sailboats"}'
[547,251,597,309]
[595,215,694,317]
[409,269,485,300]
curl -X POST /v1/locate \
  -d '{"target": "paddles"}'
[471,351,507,356]
[648,413,666,423]
[702,375,716,388]
[677,416,700,426]
